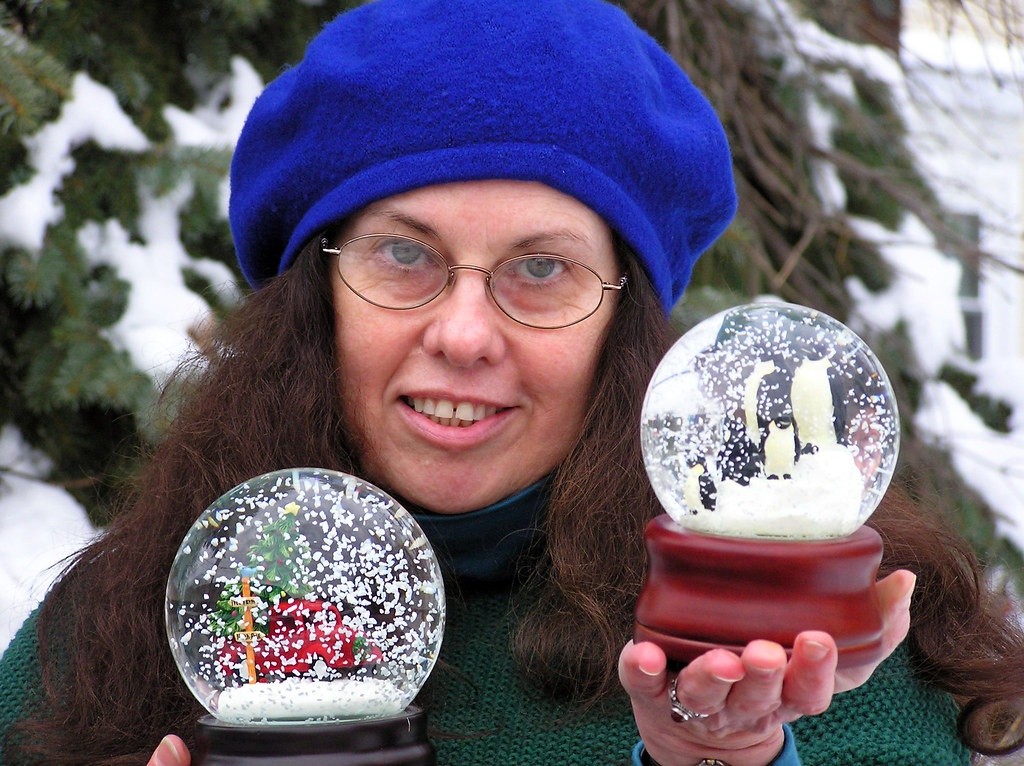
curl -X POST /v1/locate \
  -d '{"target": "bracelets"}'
[696,759,728,766]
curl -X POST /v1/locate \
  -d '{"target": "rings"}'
[667,678,710,723]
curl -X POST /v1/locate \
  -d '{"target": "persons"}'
[1,0,1022,766]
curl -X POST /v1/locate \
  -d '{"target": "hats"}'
[229,0,742,321]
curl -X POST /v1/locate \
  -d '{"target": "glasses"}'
[318,229,630,330]
